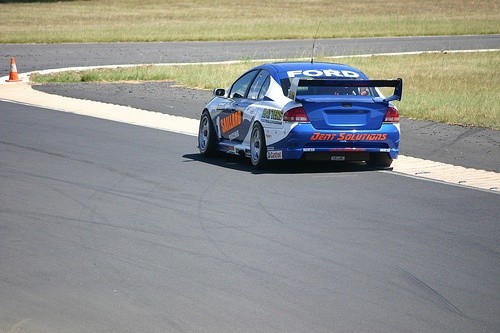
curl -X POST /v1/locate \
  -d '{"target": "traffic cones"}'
[6,57,20,82]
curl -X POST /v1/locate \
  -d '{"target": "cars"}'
[198,62,403,166]
